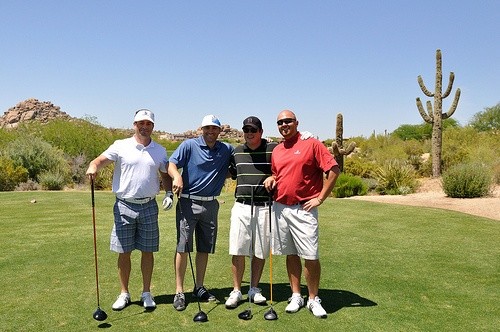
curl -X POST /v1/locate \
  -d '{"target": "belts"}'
[117,196,154,204]
[295,200,308,205]
[236,199,273,206]
[180,193,216,201]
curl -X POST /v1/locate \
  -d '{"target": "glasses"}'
[243,128,257,133]
[277,118,296,125]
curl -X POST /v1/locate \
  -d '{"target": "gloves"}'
[300,131,315,140]
[162,191,174,211]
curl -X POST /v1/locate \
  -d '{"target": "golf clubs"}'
[178,197,209,324]
[91,177,108,322]
[237,184,255,319]
[263,191,279,320]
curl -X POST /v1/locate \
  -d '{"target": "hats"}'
[242,116,262,129]
[202,115,221,127]
[133,109,155,124]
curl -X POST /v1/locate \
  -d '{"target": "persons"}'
[86,108,174,311]
[165,113,274,311]
[263,110,340,319]
[225,116,314,318]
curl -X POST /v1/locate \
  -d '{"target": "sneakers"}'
[307,295,327,318]
[174,293,185,310]
[140,292,156,308]
[285,295,305,313]
[225,290,242,307]
[248,287,266,304]
[112,293,131,310]
[192,286,216,301]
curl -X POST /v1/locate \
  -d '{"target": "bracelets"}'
[317,197,323,203]
